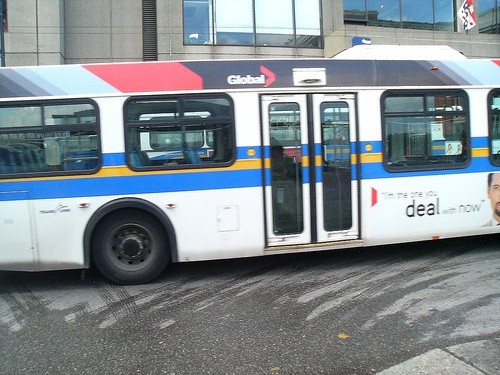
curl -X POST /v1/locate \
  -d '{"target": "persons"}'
[481,173,500,227]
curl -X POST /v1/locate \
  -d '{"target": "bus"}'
[0,42,500,286]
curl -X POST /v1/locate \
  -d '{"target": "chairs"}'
[61,149,99,170]
[132,147,204,165]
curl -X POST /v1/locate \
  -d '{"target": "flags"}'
[457,0,476,34]
